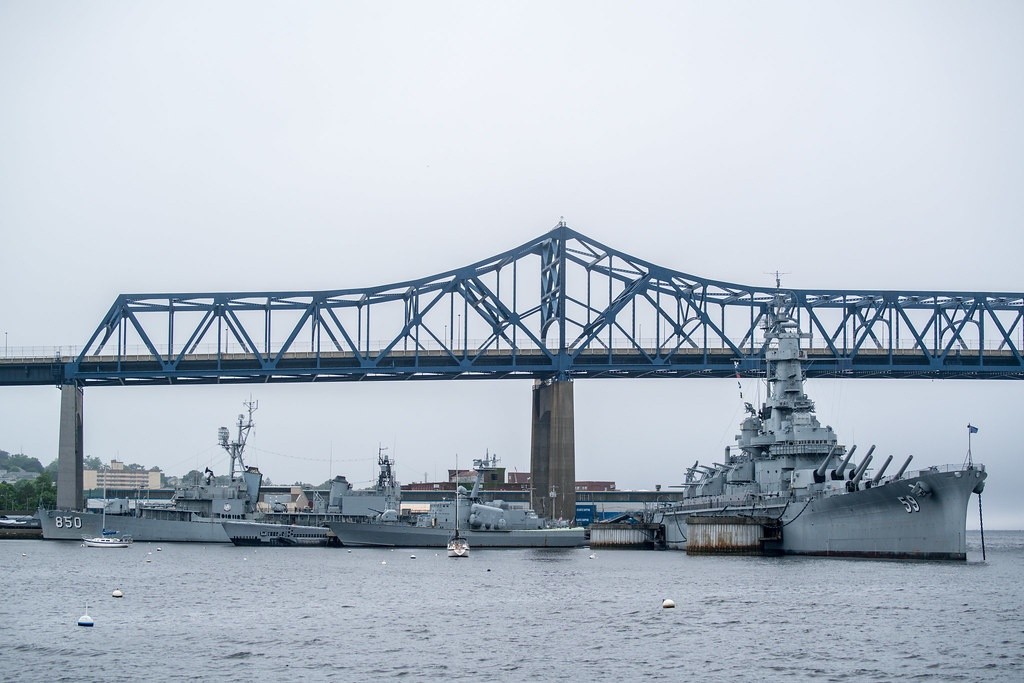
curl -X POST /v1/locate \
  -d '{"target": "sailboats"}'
[81,465,131,548]
[447,453,470,557]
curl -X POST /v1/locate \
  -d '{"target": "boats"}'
[37,396,592,547]
[650,264,991,562]
